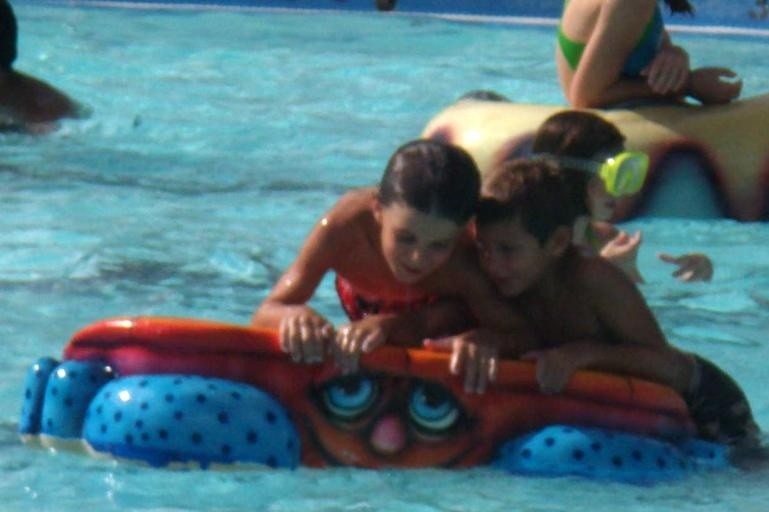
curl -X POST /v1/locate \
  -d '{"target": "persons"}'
[535,111,716,286]
[250,140,545,399]
[554,0,745,114]
[0,1,73,122]
[334,155,756,442]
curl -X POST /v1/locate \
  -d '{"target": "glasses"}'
[536,152,650,196]
